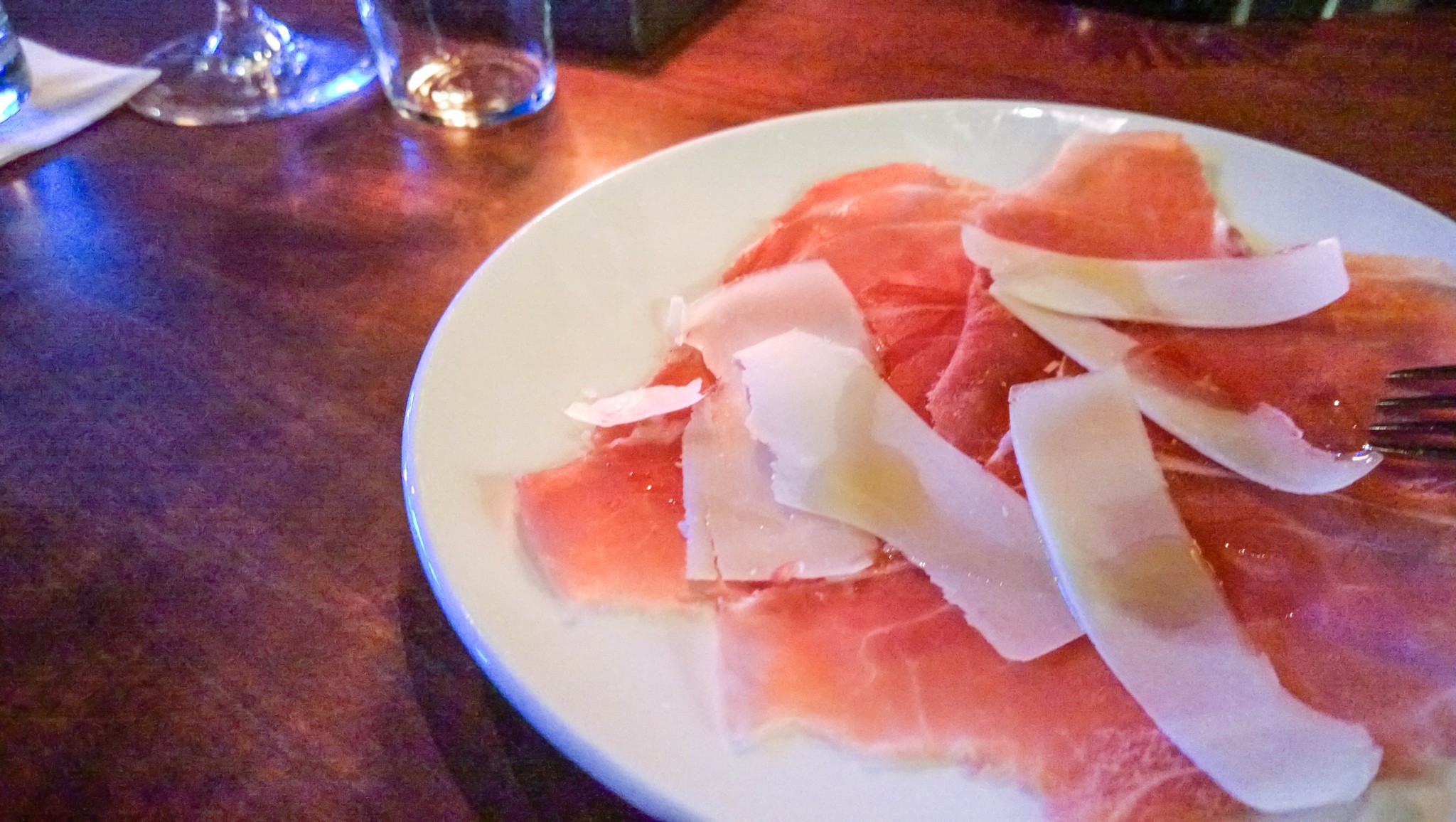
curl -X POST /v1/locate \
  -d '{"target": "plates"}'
[398,99,1456,818]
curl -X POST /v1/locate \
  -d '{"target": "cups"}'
[350,0,560,128]
